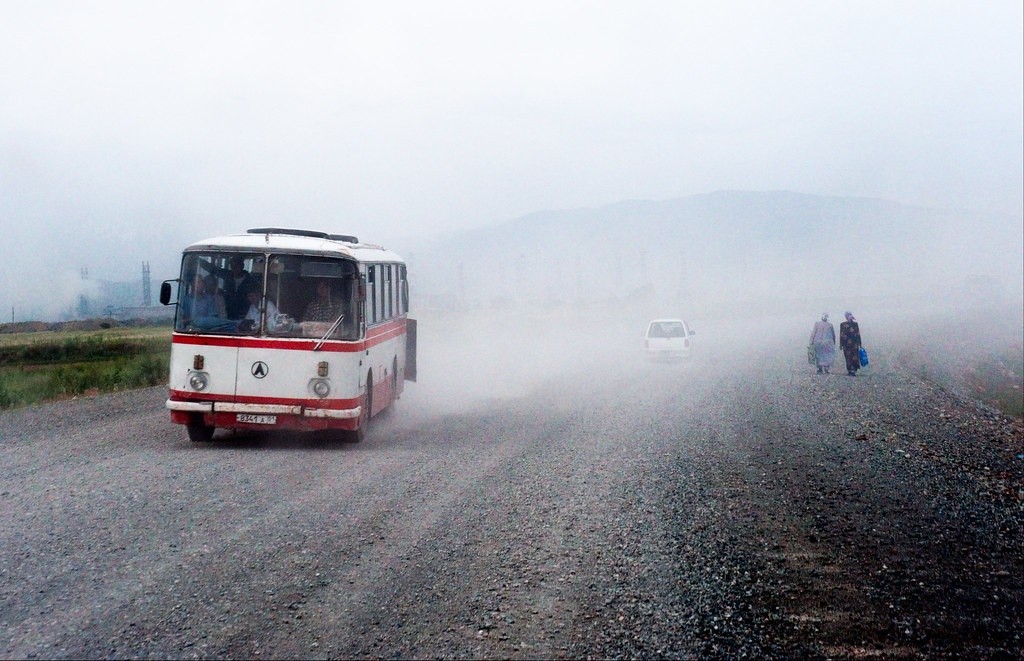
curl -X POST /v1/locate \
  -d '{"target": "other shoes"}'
[816,368,823,374]
[848,370,856,375]
[824,369,829,374]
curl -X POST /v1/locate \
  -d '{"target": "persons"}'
[198,255,260,319]
[180,275,227,331]
[809,313,836,375]
[241,286,281,333]
[300,282,344,328]
[840,312,862,376]
[202,275,233,319]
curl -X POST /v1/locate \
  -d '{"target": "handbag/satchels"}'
[859,347,868,367]
[807,344,816,365]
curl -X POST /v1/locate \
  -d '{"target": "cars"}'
[641,318,696,363]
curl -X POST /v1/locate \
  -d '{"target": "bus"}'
[159,228,419,447]
